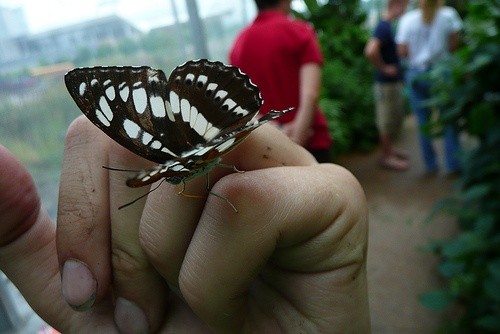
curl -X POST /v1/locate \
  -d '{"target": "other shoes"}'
[382,146,413,170]
[420,172,440,181]
[437,172,462,187]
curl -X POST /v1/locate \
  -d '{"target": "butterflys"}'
[62,57,295,214]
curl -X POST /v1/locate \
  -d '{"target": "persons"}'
[394,0,466,180]
[363,0,412,172]
[230,0,330,166]
